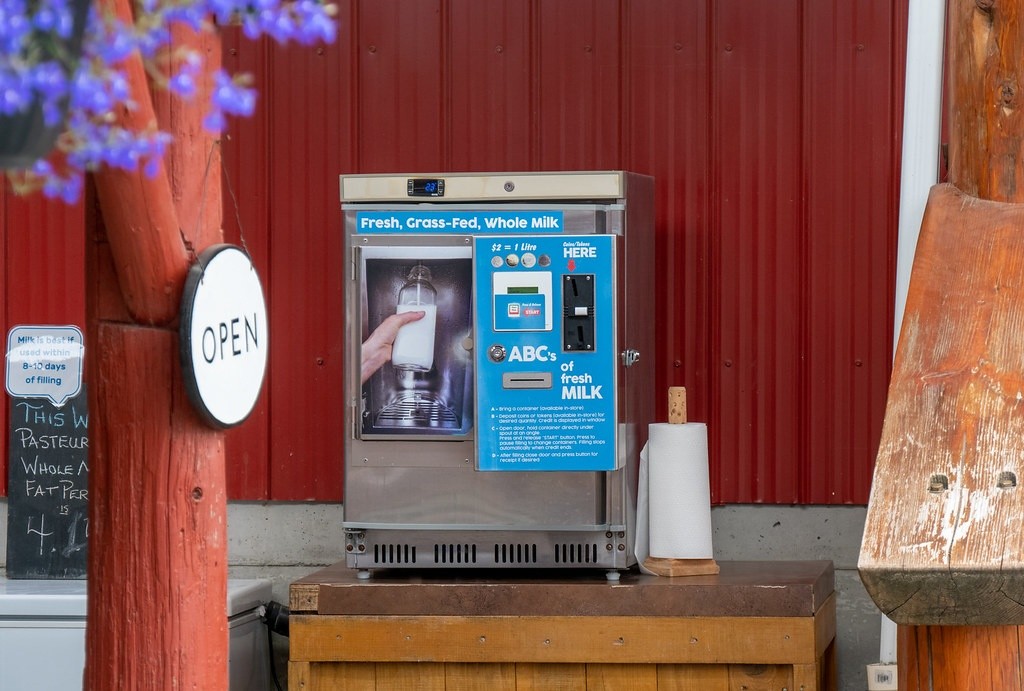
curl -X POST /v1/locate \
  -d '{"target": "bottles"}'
[391,265,436,372]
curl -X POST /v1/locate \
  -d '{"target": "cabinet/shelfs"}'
[287,590,837,691]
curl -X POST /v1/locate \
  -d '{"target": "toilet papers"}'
[634,421,714,561]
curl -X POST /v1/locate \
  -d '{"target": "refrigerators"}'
[1,575,272,691]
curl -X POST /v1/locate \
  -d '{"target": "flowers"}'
[0,0,343,210]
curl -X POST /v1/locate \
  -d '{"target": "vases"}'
[0,61,73,171]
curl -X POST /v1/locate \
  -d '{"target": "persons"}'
[361,311,425,386]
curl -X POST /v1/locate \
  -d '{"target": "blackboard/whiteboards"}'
[6,383,89,580]
